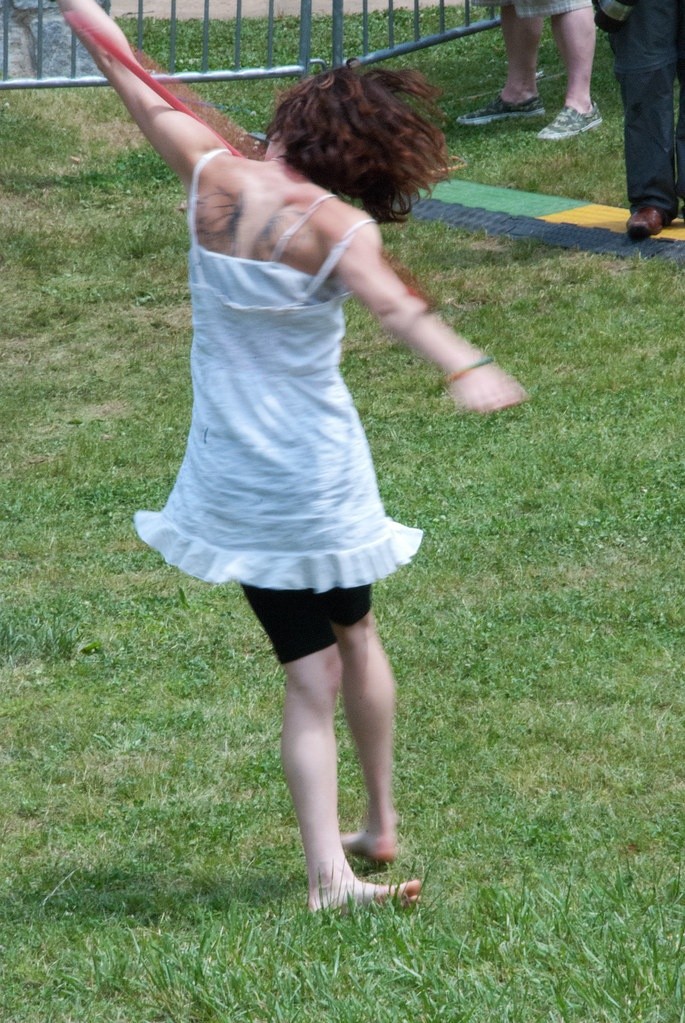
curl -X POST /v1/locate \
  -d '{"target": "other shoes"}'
[455,95,546,124]
[539,99,603,139]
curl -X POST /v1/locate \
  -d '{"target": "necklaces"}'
[271,157,283,164]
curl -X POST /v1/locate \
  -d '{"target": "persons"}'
[54,0,533,915]
[457,0,603,140]
[592,0,685,235]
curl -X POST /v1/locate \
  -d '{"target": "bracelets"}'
[447,357,495,387]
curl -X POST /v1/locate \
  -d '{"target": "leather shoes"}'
[626,210,664,233]
